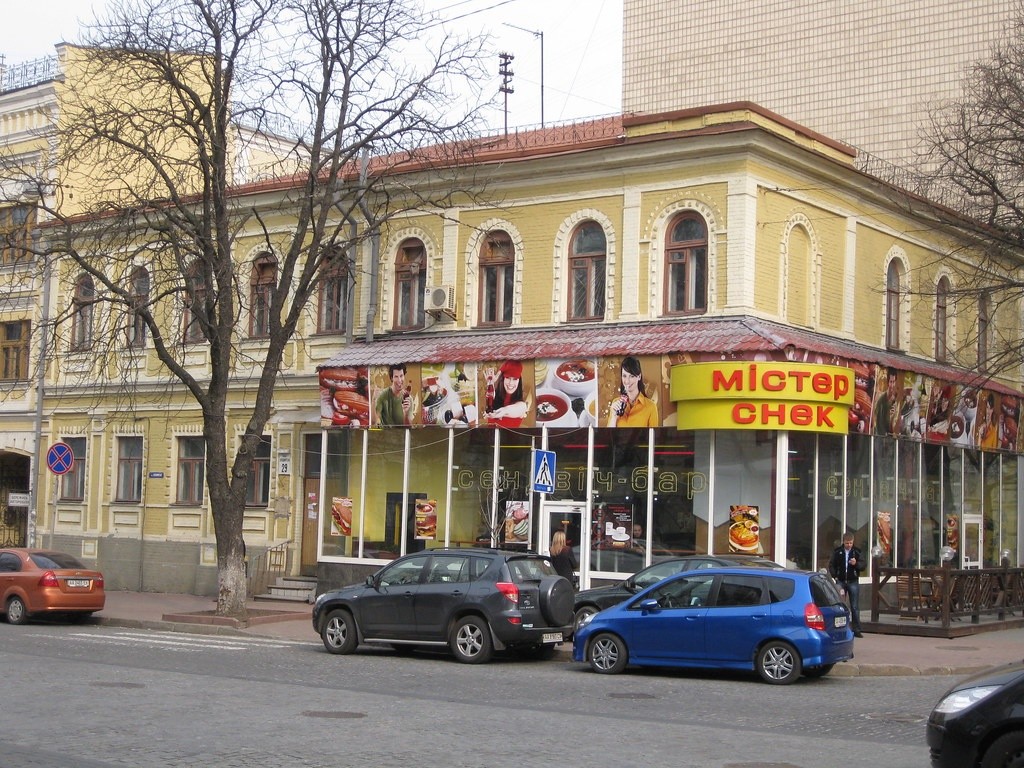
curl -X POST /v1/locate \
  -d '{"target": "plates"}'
[612,534,630,541]
[729,522,758,551]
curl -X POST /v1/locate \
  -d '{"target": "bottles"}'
[402,380,412,405]
[616,386,629,417]
[487,376,495,413]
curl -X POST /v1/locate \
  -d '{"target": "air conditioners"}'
[424,285,454,311]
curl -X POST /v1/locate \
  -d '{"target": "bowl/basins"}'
[423,387,449,425]
[439,389,475,427]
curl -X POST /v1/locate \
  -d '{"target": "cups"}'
[584,390,595,424]
[610,526,626,539]
[949,410,967,440]
[415,503,433,516]
[535,388,572,427]
[961,395,978,420]
[553,358,595,397]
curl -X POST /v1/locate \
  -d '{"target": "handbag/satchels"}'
[573,571,580,592]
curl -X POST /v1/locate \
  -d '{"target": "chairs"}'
[895,565,1024,623]
[463,559,486,580]
[430,563,449,582]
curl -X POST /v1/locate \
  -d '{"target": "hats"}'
[500,359,522,377]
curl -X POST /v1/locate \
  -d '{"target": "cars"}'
[1,546,107,626]
[927,659,1024,768]
[570,565,855,685]
[576,559,787,645]
[311,544,575,665]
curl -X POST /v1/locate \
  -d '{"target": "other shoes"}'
[854,632,863,638]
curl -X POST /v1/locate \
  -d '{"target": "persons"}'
[930,384,951,427]
[605,357,659,427]
[482,359,528,428]
[550,531,579,585]
[375,365,415,427]
[829,532,867,638]
[977,393,997,451]
[633,524,643,539]
[875,367,900,436]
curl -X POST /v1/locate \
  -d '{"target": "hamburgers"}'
[319,368,370,419]
[331,503,352,534]
[877,517,892,552]
